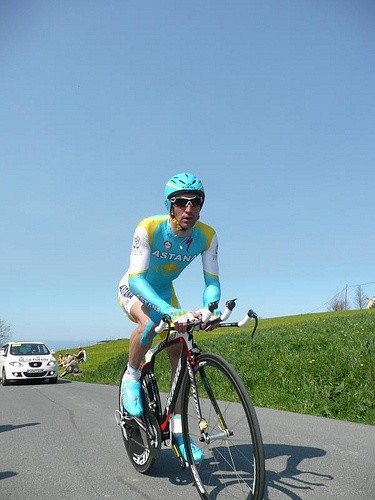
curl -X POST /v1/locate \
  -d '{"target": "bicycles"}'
[116,299,266,500]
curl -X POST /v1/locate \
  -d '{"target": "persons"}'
[57,346,86,378]
[118,173,222,464]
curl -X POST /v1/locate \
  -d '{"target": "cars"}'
[0,341,58,385]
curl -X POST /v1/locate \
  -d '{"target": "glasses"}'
[170,196,203,207]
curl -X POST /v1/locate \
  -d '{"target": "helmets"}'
[162,172,206,212]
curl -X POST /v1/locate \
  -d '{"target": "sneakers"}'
[121,373,144,417]
[172,435,204,465]
